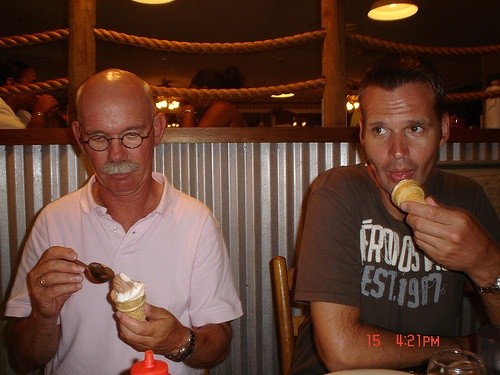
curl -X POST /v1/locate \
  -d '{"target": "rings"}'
[40,278,46,287]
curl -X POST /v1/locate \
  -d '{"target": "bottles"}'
[486,80,500,128]
[130,350,171,375]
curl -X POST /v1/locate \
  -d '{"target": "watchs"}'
[477,276,500,293]
[165,327,195,362]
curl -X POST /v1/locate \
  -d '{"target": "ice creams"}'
[110,272,148,320]
[392,179,428,207]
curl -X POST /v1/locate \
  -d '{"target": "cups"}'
[427,348,487,375]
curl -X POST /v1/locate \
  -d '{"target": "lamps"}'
[346,94,360,110]
[367,0,418,22]
[154,57,180,110]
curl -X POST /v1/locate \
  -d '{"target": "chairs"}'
[270,255,308,375]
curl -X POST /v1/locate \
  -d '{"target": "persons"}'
[177,68,246,128]
[4,69,244,375]
[287,64,500,375]
[0,61,59,129]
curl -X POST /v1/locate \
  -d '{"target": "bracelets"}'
[181,110,191,117]
[34,112,44,116]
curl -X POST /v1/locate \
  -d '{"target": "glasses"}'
[80,121,154,151]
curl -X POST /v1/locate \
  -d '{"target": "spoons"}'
[73,259,115,284]
